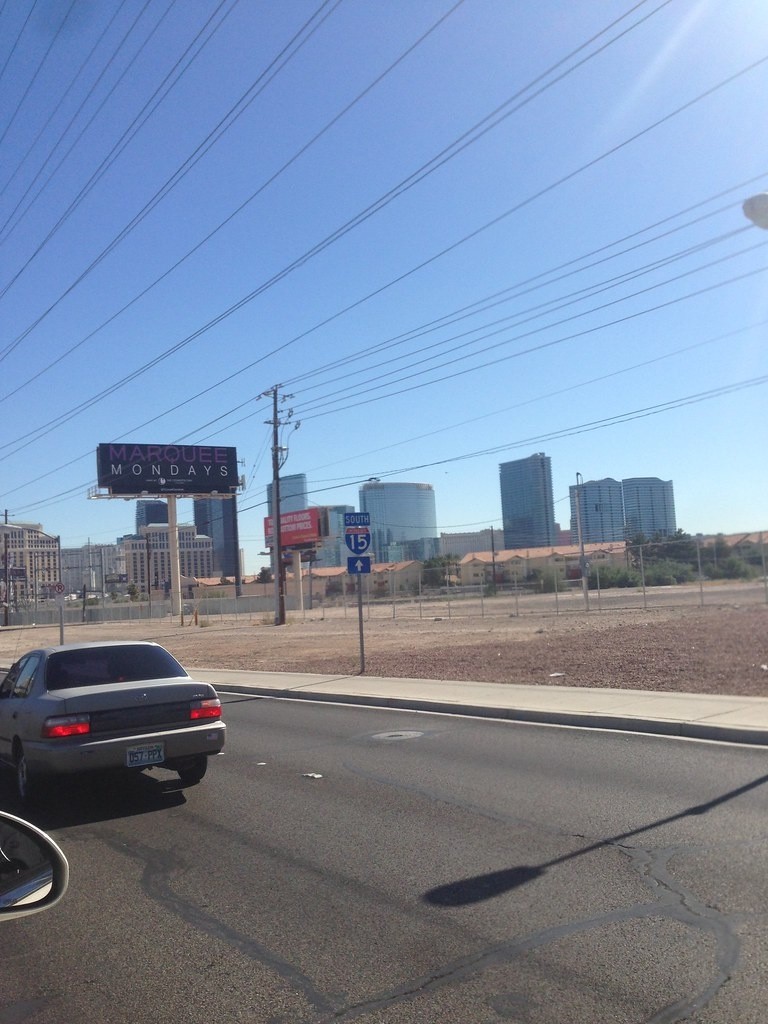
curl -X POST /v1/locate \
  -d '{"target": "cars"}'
[0,640,226,806]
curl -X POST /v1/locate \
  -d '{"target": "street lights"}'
[270,447,290,625]
